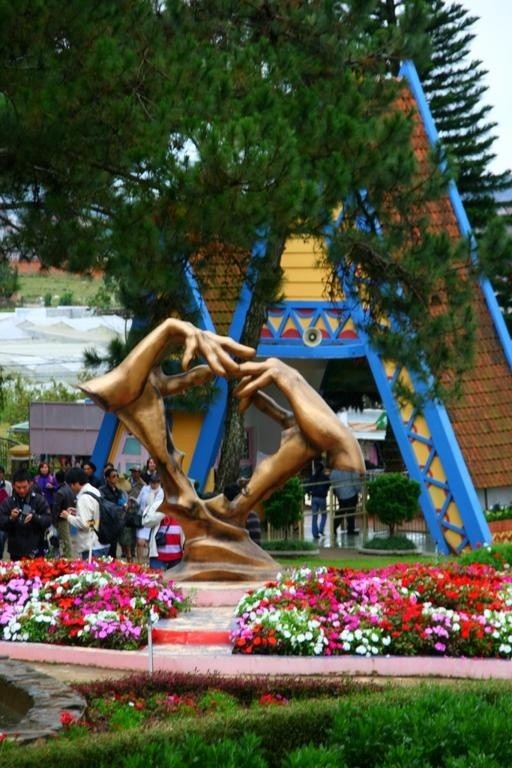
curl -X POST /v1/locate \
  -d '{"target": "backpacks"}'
[81,488,129,546]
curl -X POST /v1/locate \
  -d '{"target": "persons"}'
[225,483,262,546]
[329,468,361,535]
[80,317,368,582]
[0,457,184,571]
[307,462,331,537]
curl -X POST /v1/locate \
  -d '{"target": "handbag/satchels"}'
[44,523,60,553]
[126,512,142,528]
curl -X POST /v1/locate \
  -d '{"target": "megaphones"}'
[303,326,322,348]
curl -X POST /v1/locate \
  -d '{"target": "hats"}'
[129,465,141,473]
